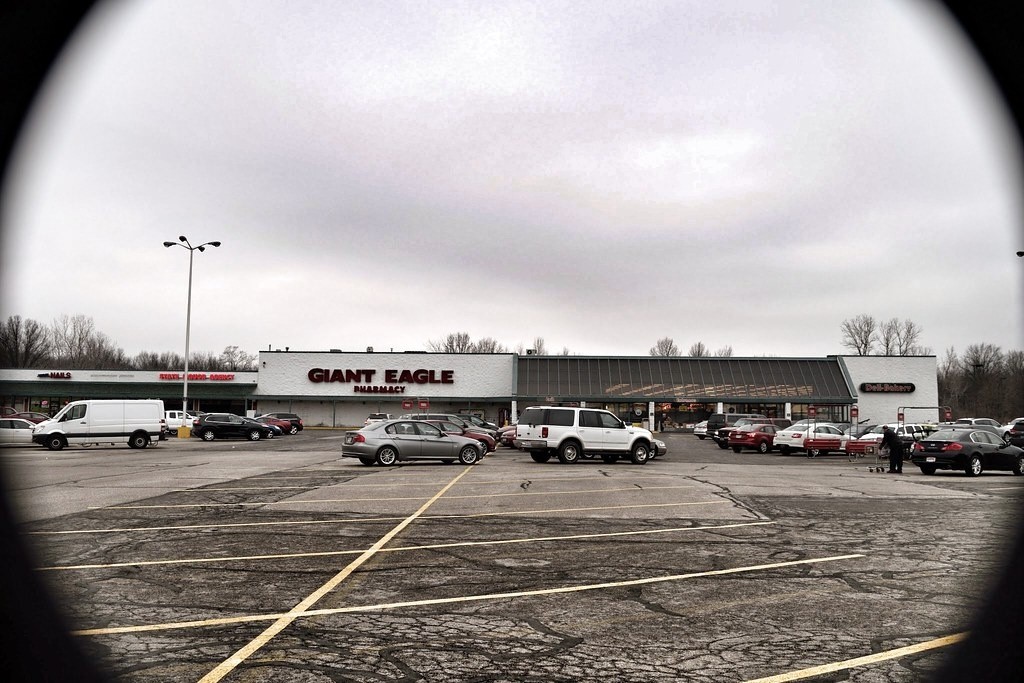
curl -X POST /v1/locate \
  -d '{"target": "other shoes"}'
[887,470,896,473]
[896,470,902,473]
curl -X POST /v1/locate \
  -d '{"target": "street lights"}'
[163,236,221,438]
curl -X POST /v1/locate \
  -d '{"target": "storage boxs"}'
[679,406,687,411]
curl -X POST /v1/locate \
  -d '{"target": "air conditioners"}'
[526,349,535,355]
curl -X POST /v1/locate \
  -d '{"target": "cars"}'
[728,417,1024,457]
[695,420,708,439]
[341,419,484,467]
[243,414,292,439]
[418,420,497,452]
[908,428,1024,478]
[498,419,518,448]
[0,406,51,447]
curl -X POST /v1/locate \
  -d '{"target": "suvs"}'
[165,410,205,436]
[364,413,394,427]
[713,418,792,449]
[399,414,498,441]
[513,406,667,464]
[193,413,270,441]
[262,412,303,435]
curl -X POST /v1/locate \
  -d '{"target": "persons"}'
[878,425,904,473]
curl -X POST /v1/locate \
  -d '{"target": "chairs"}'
[400,425,405,431]
[407,427,413,432]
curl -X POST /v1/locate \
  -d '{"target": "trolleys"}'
[867,443,885,473]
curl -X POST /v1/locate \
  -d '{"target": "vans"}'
[706,413,768,443]
[32,399,167,450]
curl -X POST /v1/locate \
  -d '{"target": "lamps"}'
[262,361,266,368]
